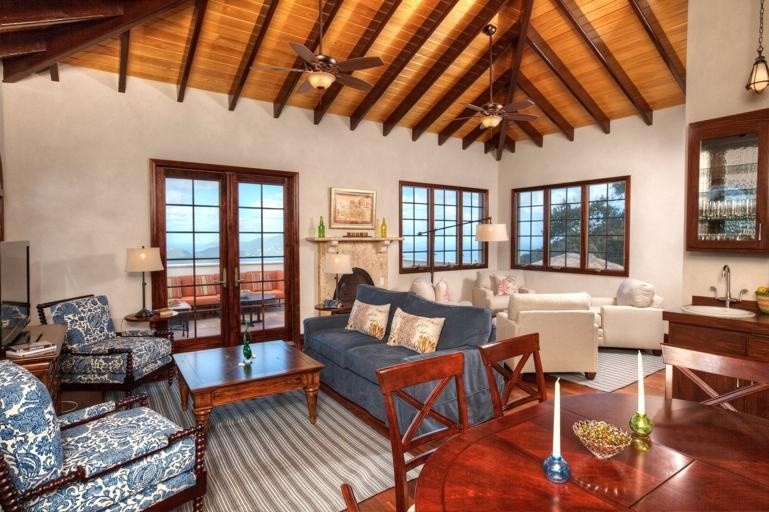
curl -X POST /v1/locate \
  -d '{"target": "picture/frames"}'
[330,188,376,229]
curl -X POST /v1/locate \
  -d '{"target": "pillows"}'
[344,298,447,355]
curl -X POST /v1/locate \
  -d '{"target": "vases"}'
[757,295,769,314]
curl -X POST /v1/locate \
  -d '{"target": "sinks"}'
[681,305,757,317]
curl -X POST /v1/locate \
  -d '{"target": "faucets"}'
[721,264,732,307]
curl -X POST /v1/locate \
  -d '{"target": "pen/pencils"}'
[27,335,31,343]
[29,344,56,350]
[35,334,43,342]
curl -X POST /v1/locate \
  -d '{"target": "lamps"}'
[307,71,337,90]
[480,115,502,130]
[126,246,164,318]
[323,252,354,307]
[418,217,509,284]
[745,0,769,94]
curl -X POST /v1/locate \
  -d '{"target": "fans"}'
[249,0,384,91]
[451,24,538,121]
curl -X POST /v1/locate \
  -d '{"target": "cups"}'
[699,198,756,219]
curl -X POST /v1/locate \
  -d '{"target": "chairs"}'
[658,341,767,413]
[374,351,470,511]
[411,269,664,380]
[0,357,206,512]
[341,482,365,512]
[477,332,550,420]
[31,294,173,409]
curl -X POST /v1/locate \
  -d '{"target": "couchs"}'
[167,269,284,318]
[303,284,504,438]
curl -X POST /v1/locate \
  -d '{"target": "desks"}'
[3,321,69,415]
[411,392,769,512]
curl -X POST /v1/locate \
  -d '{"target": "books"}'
[5,345,59,358]
[6,339,55,352]
[154,308,172,316]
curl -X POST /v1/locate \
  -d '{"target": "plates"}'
[571,418,632,460]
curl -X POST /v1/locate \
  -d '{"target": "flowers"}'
[755,287,769,296]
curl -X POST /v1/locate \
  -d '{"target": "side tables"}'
[315,304,353,316]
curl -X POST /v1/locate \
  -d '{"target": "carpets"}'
[108,379,425,512]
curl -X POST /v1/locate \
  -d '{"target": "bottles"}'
[380,216,387,238]
[317,216,325,238]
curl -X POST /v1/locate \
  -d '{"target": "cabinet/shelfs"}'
[686,108,769,253]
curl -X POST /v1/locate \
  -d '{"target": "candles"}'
[548,378,566,459]
[633,350,651,417]
